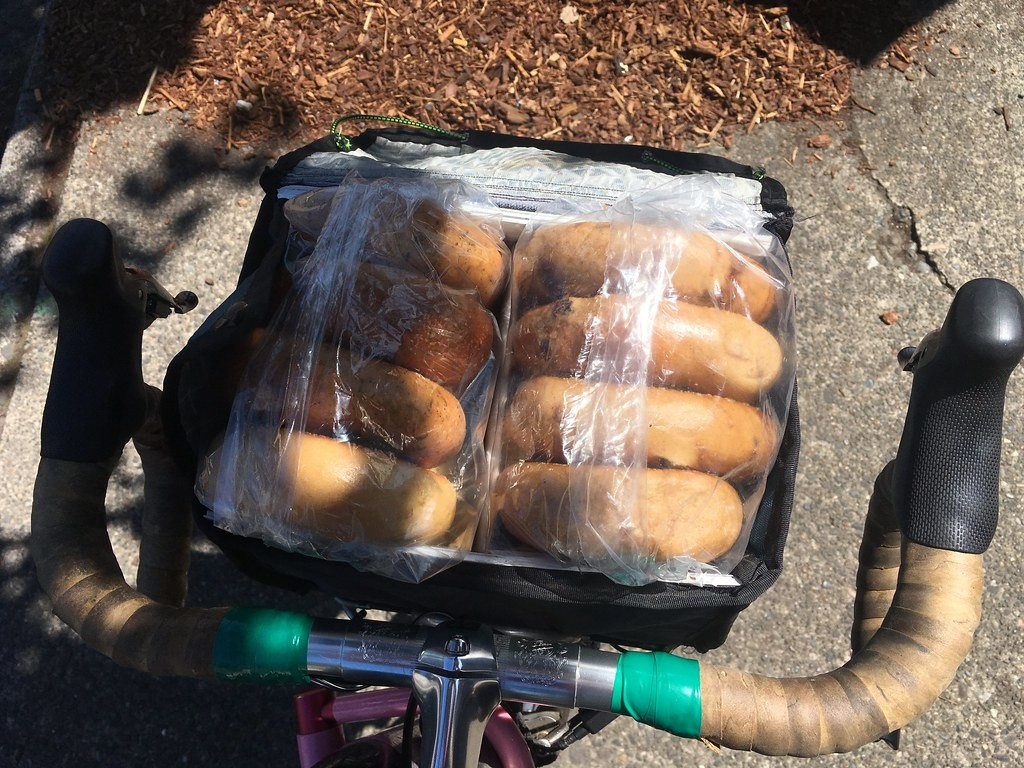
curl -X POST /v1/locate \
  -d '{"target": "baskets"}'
[159,129,798,651]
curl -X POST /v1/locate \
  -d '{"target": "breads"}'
[490,217,781,565]
[200,187,509,545]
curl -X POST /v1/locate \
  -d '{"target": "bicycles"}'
[28,127,1024,768]
